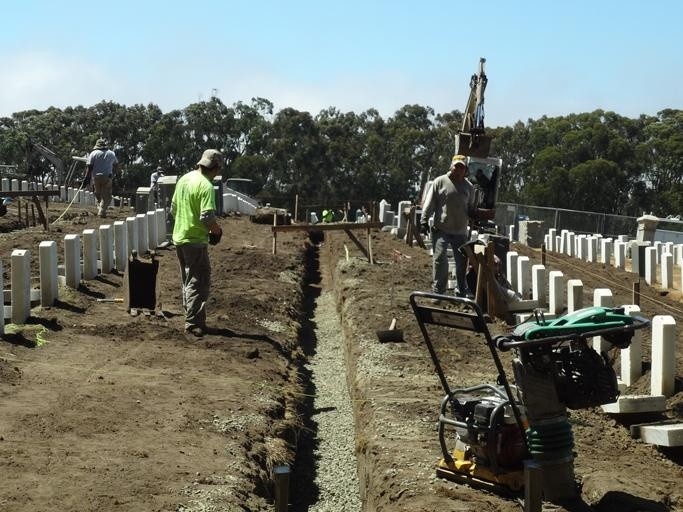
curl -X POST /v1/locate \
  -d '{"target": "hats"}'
[196,148,224,169]
[451,154,467,166]
[93,139,108,149]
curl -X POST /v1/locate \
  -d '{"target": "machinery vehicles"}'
[419,57,502,233]
[23,134,95,193]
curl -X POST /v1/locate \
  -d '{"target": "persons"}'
[150,167,165,201]
[83,138,119,216]
[169,147,223,337]
[419,154,484,305]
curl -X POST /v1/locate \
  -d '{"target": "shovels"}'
[376,317,404,344]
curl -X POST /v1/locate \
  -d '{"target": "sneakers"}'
[455,288,474,298]
[431,291,442,303]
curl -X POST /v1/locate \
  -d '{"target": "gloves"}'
[83,177,89,184]
[420,223,430,236]
[208,228,222,245]
